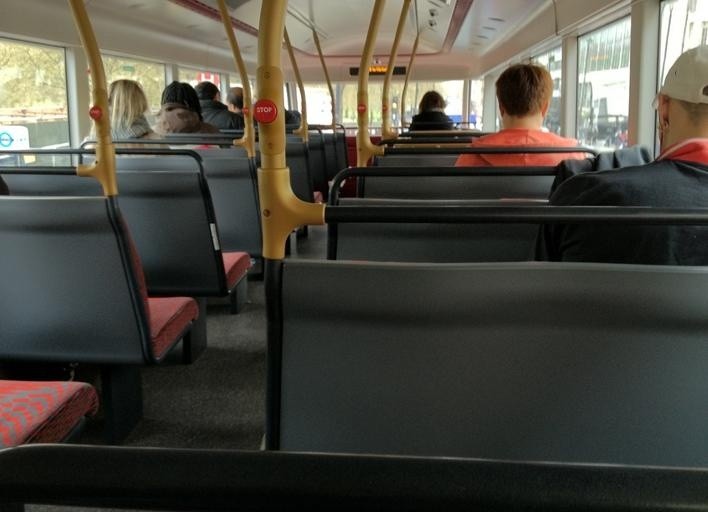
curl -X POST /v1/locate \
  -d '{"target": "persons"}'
[454,61,585,167]
[82,80,170,158]
[530,41,707,267]
[410,90,455,143]
[225,87,243,115]
[546,120,629,150]
[191,82,244,132]
[285,107,301,123]
[151,80,226,149]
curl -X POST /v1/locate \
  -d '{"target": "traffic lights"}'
[390,96,400,123]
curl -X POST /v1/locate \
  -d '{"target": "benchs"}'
[0,122,707,512]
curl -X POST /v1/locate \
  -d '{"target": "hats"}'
[650,44,708,111]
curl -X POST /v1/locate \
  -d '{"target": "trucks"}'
[542,68,632,136]
[0,124,39,177]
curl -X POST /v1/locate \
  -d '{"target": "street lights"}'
[580,35,598,143]
